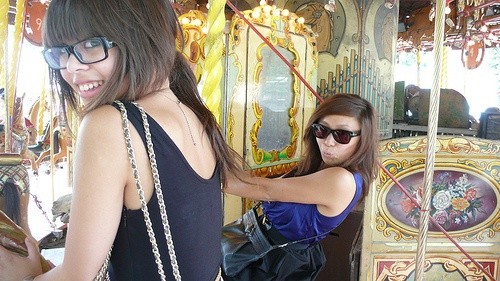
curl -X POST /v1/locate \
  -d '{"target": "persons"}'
[223,93,378,281]
[0,0,242,281]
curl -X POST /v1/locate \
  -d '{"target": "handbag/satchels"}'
[220,208,273,277]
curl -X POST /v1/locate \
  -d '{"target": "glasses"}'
[310,124,361,145]
[41,35,117,71]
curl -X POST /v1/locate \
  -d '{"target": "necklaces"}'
[137,89,195,146]
